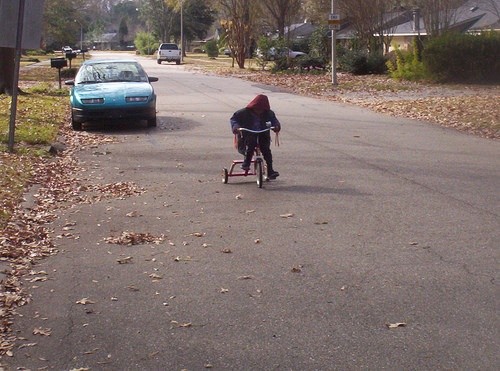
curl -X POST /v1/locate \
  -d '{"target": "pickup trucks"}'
[158,43,182,65]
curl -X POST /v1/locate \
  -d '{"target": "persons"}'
[229,94,281,181]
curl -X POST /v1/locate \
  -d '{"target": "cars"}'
[64,59,159,130]
[259,47,307,60]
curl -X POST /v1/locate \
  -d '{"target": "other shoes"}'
[267,170,279,177]
[241,162,250,169]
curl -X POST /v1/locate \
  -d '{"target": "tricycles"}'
[221,125,276,188]
